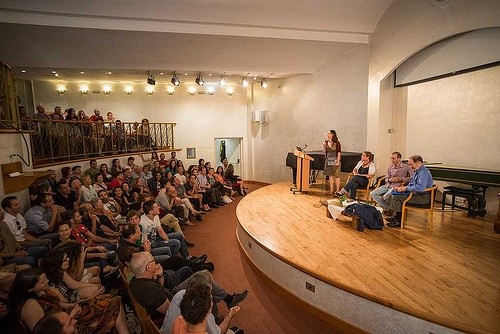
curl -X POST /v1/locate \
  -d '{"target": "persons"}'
[384,154,434,227]
[334,151,376,203]
[322,129,342,197]
[369,151,414,216]
[407,157,414,171]
[0,104,251,334]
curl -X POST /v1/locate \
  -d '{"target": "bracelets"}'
[112,231,115,234]
[158,274,164,278]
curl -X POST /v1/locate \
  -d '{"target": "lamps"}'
[147,70,155,86]
[102,85,111,95]
[261,79,267,88]
[145,85,154,95]
[207,87,215,95]
[124,86,133,95]
[188,87,196,95]
[80,85,89,95]
[170,71,180,86]
[166,86,174,95]
[226,86,233,97]
[195,72,205,87]
[56,84,66,95]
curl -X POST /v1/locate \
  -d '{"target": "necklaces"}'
[363,161,371,168]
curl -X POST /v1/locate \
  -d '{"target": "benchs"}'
[442,186,483,217]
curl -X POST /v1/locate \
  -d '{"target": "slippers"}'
[104,265,120,278]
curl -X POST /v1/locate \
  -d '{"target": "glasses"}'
[16,221,21,231]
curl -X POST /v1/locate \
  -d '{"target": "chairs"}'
[118,269,149,334]
[373,176,408,206]
[143,314,162,334]
[391,184,438,232]
[345,173,375,205]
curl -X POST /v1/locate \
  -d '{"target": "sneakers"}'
[226,288,249,310]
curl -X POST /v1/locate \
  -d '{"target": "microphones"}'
[301,143,308,151]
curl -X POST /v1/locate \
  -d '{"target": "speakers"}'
[252,111,260,122]
[260,110,269,125]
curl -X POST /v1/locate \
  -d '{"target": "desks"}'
[401,161,500,217]
[320,198,360,230]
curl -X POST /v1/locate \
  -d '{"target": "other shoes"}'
[207,206,212,211]
[216,202,224,206]
[184,239,195,248]
[190,254,207,264]
[184,220,195,226]
[334,191,345,197]
[185,255,199,262]
[210,204,219,208]
[192,211,203,216]
[386,216,397,222]
[387,218,402,227]
[196,215,202,221]
[203,206,209,211]
[323,192,334,196]
